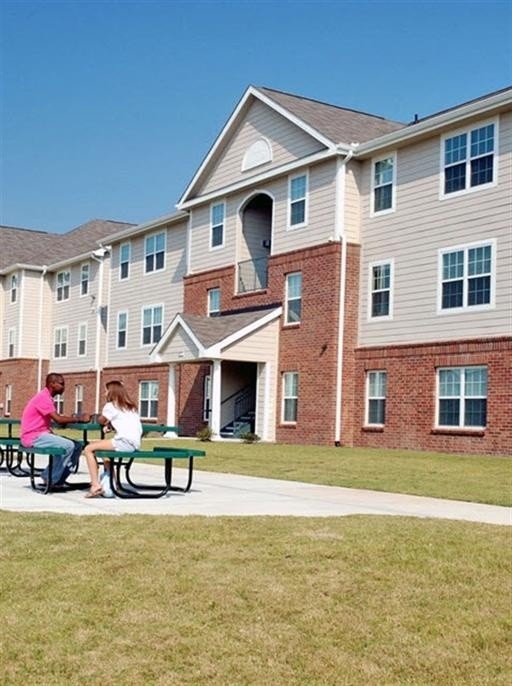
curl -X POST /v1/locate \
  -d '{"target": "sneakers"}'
[44,480,71,491]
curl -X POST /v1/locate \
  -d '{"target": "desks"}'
[0,417,184,441]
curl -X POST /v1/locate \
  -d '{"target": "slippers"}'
[84,488,105,498]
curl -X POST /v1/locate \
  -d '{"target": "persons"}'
[83,380,144,498]
[20,373,96,492]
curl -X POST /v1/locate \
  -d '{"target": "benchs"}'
[0,436,206,502]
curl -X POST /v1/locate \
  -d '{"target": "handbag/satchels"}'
[99,471,116,499]
